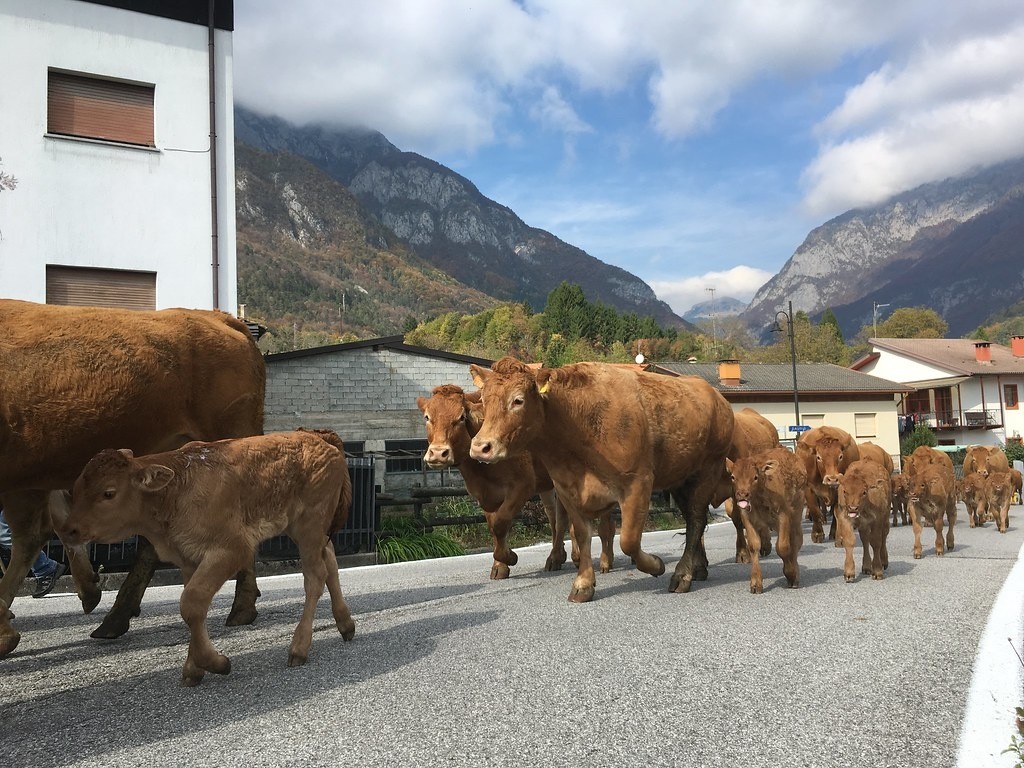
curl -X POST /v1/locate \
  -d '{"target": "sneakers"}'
[32,563,66,598]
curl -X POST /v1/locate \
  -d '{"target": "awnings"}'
[930,445,980,452]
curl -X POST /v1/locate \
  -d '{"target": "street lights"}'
[770,301,801,441]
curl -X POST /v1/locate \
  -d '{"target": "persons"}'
[0,506,66,598]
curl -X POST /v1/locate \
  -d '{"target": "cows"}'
[416,355,1024,604]
[0,298,353,690]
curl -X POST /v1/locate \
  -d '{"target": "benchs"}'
[965,412,993,426]
